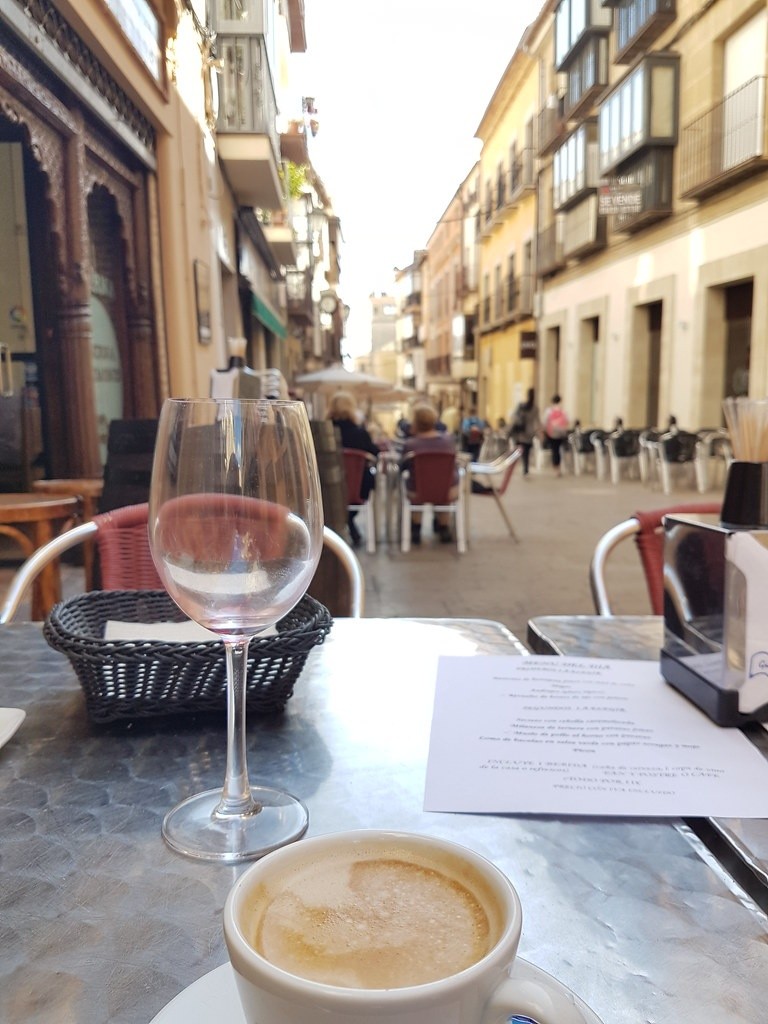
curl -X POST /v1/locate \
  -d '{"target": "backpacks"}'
[469,419,481,445]
[546,408,567,439]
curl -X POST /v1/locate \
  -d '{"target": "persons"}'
[323,388,570,546]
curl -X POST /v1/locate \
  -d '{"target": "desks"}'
[524,614,768,905]
[354,442,416,545]
[0,491,85,621]
[0,617,768,1024]
[32,478,103,605]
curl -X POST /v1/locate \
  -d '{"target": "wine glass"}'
[147,397,327,864]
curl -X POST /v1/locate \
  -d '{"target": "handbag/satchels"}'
[512,417,527,433]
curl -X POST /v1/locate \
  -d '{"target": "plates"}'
[148,957,605,1024]
[0,707,27,750]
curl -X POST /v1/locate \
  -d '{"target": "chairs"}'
[0,414,753,623]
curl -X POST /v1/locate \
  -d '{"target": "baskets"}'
[42,587,333,719]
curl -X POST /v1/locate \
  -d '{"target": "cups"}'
[222,830,523,1024]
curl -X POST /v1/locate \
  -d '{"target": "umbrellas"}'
[295,361,416,401]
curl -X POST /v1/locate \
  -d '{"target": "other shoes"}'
[433,517,452,544]
[413,523,422,544]
